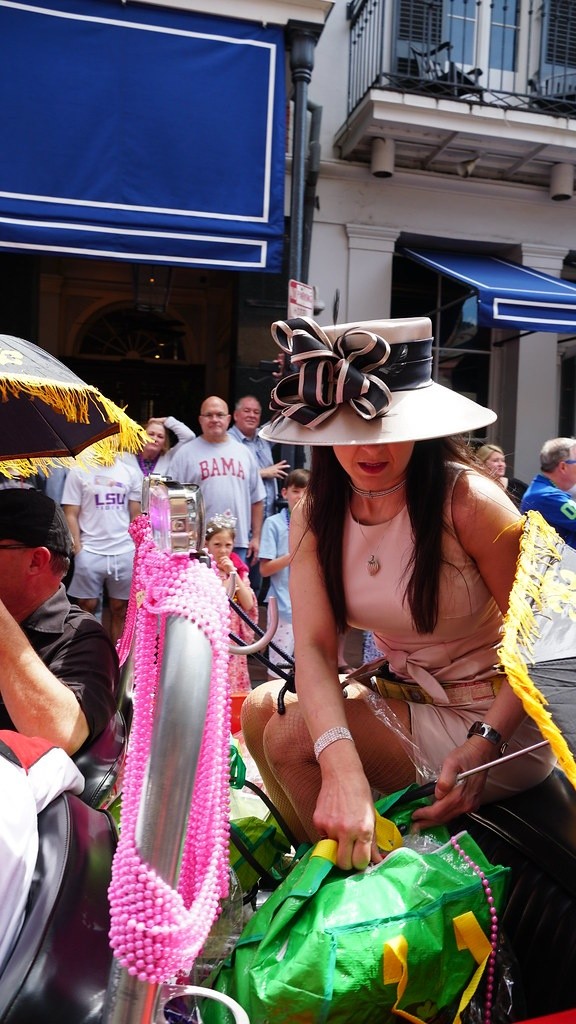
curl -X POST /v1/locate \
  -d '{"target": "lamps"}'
[371,138,395,178]
[550,164,576,201]
[129,262,175,313]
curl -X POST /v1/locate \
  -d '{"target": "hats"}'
[256,316,498,446]
[0,488,72,555]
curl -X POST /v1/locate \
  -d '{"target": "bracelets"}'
[314,727,354,765]
[466,721,509,758]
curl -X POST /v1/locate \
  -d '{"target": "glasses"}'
[201,412,228,419]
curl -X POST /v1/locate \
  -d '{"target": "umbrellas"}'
[0,334,154,480]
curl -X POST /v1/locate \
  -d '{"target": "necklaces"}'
[348,480,406,577]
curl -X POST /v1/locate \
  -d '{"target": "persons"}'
[59,434,144,647]
[259,469,310,681]
[205,509,253,610]
[168,396,267,567]
[241,317,558,868]
[0,487,120,758]
[519,438,576,549]
[228,396,291,516]
[129,417,195,476]
[476,444,529,509]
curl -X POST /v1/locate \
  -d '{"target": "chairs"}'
[408,38,484,101]
[528,79,576,115]
[1,515,234,1024]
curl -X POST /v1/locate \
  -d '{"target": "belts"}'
[374,668,495,706]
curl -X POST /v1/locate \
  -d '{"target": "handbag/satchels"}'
[201,783,512,1024]
[227,745,291,900]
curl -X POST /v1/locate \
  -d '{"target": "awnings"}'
[399,242,576,335]
